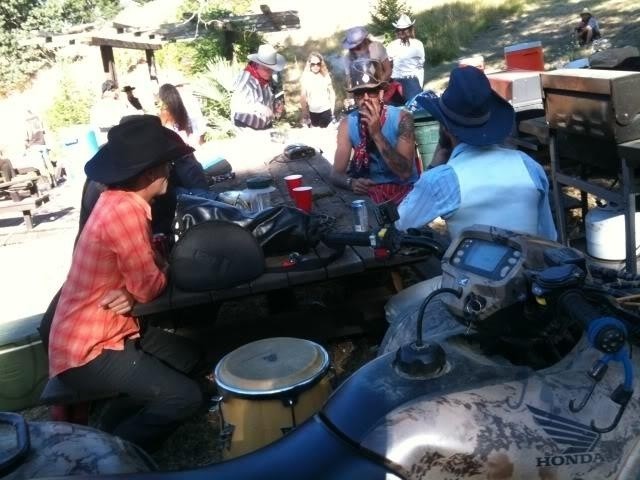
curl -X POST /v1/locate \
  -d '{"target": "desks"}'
[131,147,425,344]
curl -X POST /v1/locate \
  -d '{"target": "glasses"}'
[310,63,322,67]
[350,90,380,98]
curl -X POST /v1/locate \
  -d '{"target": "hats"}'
[415,65,514,146]
[390,12,416,30]
[342,59,390,94]
[247,45,286,71]
[85,115,194,185]
[342,27,368,49]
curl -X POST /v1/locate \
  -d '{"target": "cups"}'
[292,186,312,213]
[284,174,302,199]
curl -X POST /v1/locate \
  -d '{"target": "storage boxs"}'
[504,40,546,74]
[538,65,640,146]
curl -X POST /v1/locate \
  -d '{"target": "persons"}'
[90,80,144,148]
[383,65,559,325]
[121,85,143,110]
[341,27,392,98]
[386,14,426,91]
[48,120,215,454]
[329,59,418,207]
[230,43,287,131]
[299,51,336,128]
[158,83,198,150]
[576,7,600,46]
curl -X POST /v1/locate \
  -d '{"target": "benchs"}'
[407,222,452,280]
[37,374,120,431]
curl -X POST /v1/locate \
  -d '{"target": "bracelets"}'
[344,176,353,190]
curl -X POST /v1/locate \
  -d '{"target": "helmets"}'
[167,221,266,293]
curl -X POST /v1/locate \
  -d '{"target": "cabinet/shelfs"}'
[517,117,640,281]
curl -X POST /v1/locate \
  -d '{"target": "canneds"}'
[350,200,369,231]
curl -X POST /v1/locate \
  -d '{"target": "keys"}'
[463,310,477,337]
